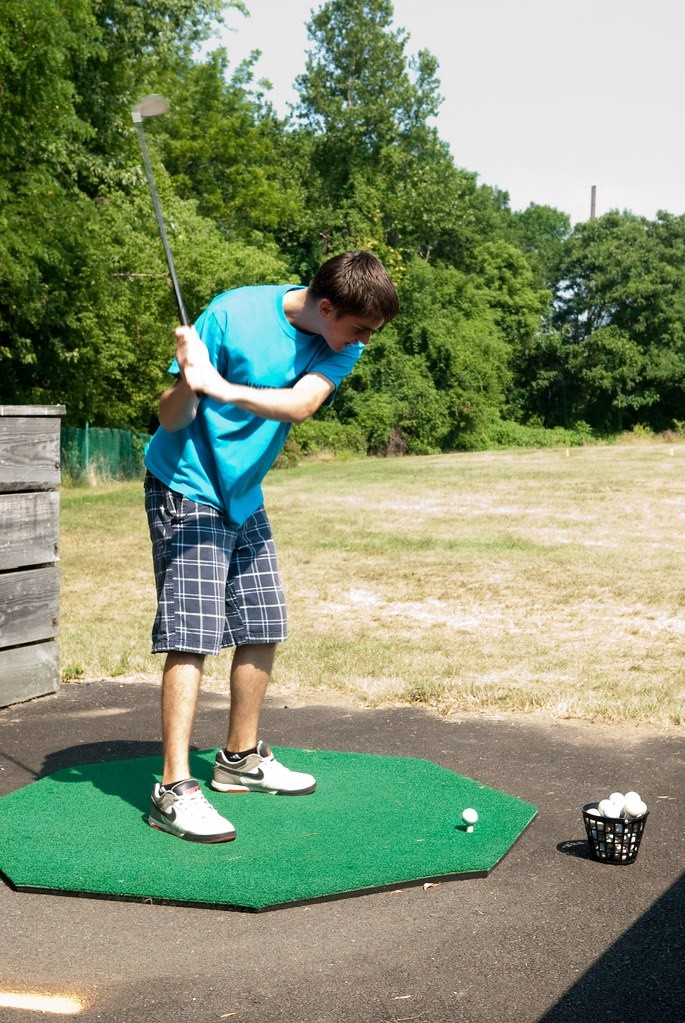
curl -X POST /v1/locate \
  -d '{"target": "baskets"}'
[582,802,649,865]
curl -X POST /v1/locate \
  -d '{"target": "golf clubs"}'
[130,93,189,324]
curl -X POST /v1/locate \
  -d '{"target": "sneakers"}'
[149,779,236,844]
[211,741,318,796]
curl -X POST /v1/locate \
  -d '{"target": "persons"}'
[141,251,401,844]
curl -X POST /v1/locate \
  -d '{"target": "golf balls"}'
[583,790,649,861]
[462,807,478,825]
